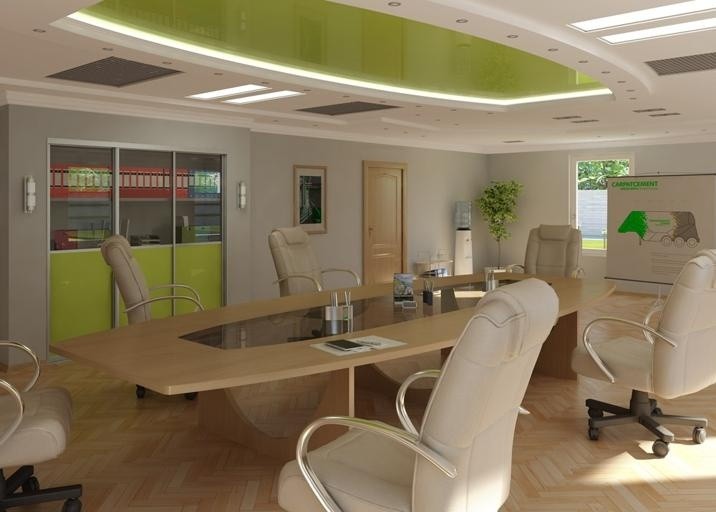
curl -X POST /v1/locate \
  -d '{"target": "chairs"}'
[276,276,559,512]
[505,224,587,278]
[101,235,204,400]
[570,246,716,457]
[269,227,362,297]
[0,339,84,511]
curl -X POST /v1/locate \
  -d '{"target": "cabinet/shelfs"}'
[44,138,226,360]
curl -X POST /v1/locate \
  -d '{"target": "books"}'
[326,339,364,351]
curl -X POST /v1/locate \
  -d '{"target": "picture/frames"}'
[292,163,328,234]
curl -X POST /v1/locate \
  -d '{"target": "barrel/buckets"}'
[455,200,472,228]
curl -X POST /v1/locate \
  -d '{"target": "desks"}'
[44,271,618,439]
